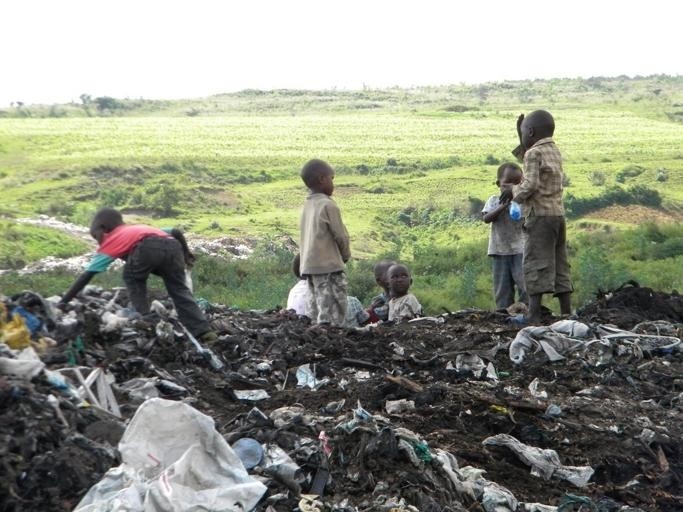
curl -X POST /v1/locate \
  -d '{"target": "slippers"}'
[509,314,528,324]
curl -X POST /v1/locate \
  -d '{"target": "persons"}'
[384,264,422,324]
[56,208,220,347]
[479,161,529,314]
[344,293,369,329]
[498,109,576,327]
[368,259,398,324]
[285,251,308,319]
[299,157,351,328]
